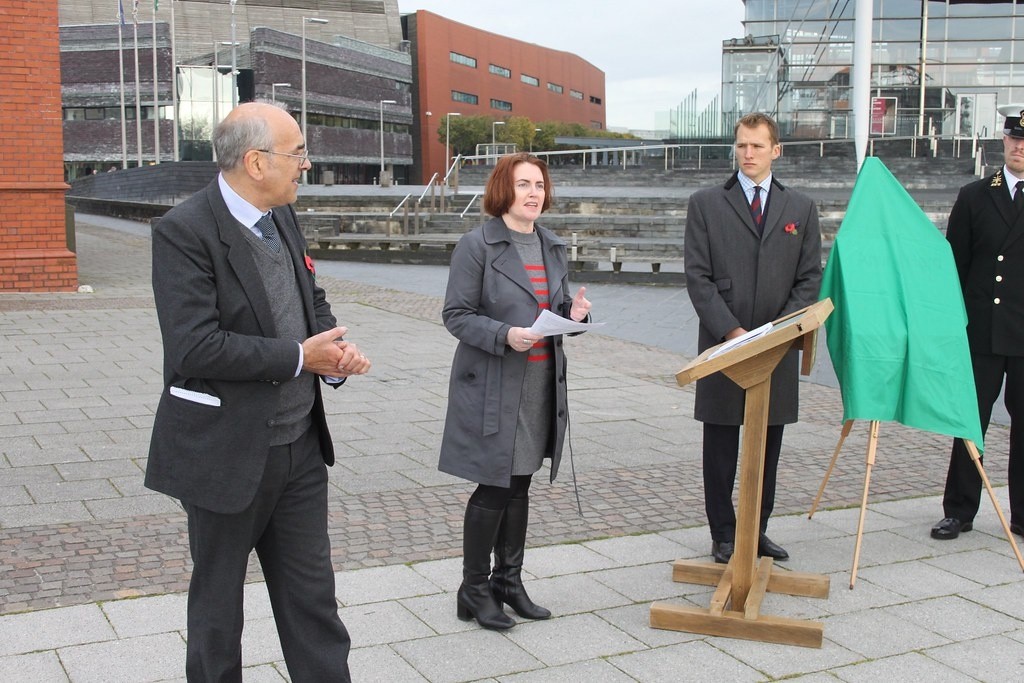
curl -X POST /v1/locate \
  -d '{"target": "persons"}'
[142,101,370,683]
[684,112,824,565]
[438,151,592,632]
[929,104,1024,541]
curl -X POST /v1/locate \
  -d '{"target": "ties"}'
[1014,181,1024,217]
[255,214,281,253]
[748,185,763,227]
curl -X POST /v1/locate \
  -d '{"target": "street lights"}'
[492,121,506,163]
[444,112,461,188]
[213,41,239,162]
[380,100,397,171]
[301,17,329,185]
[529,128,541,153]
[273,82,292,104]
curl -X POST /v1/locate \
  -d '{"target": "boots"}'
[490,498,552,621]
[457,499,517,629]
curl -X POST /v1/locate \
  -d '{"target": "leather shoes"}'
[712,539,735,564]
[1011,523,1024,537]
[758,534,789,560]
[930,516,972,540]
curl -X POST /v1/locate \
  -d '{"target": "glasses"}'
[256,148,308,167]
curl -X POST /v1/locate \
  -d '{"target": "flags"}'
[120,0,158,29]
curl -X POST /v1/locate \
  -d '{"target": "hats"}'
[997,104,1024,138]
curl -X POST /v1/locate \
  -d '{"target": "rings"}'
[524,340,527,344]
